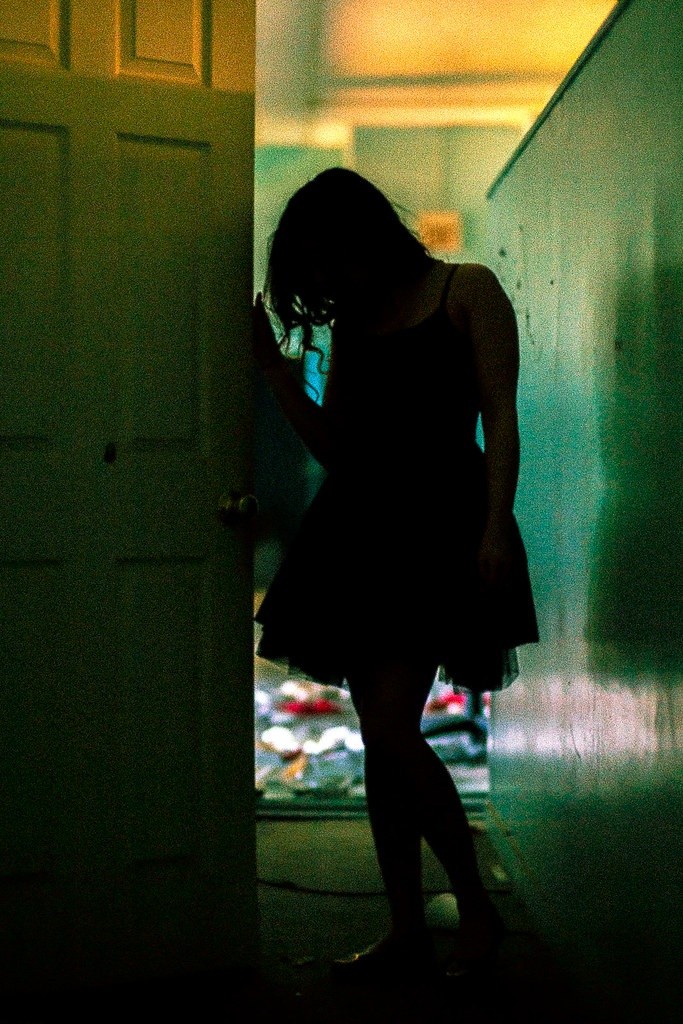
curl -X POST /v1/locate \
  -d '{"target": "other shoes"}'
[332,933,439,971]
[444,916,508,966]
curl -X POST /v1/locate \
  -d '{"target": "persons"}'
[252,168,538,979]
